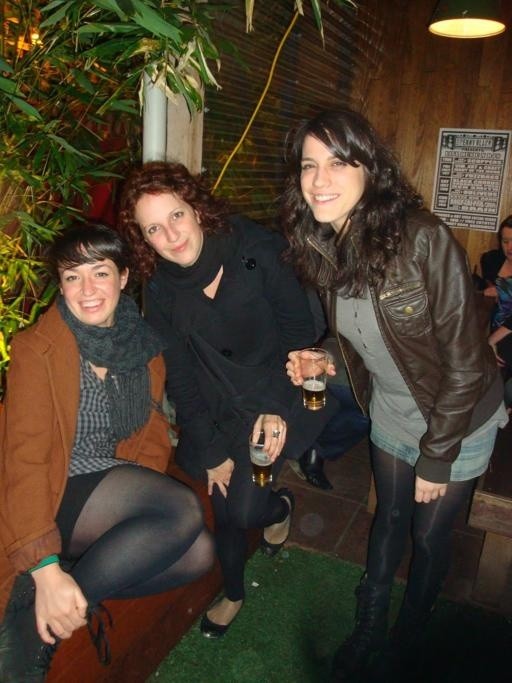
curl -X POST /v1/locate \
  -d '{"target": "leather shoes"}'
[286,447,334,490]
[201,596,244,639]
[261,487,294,561]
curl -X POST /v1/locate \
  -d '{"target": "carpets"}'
[147,537,512,682]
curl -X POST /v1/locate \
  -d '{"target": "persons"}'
[117,155,330,644]
[0,213,222,683]
[471,213,512,369]
[285,103,512,679]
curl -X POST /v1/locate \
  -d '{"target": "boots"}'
[367,588,435,683]
[331,577,392,670]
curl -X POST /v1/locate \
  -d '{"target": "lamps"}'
[417,0,512,43]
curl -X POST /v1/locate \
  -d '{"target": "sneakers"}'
[0,573,85,683]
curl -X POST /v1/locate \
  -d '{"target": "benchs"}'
[40,447,274,681]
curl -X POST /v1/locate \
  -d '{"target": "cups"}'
[249,430,279,489]
[296,347,329,412]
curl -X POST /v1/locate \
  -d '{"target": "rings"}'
[273,429,281,438]
